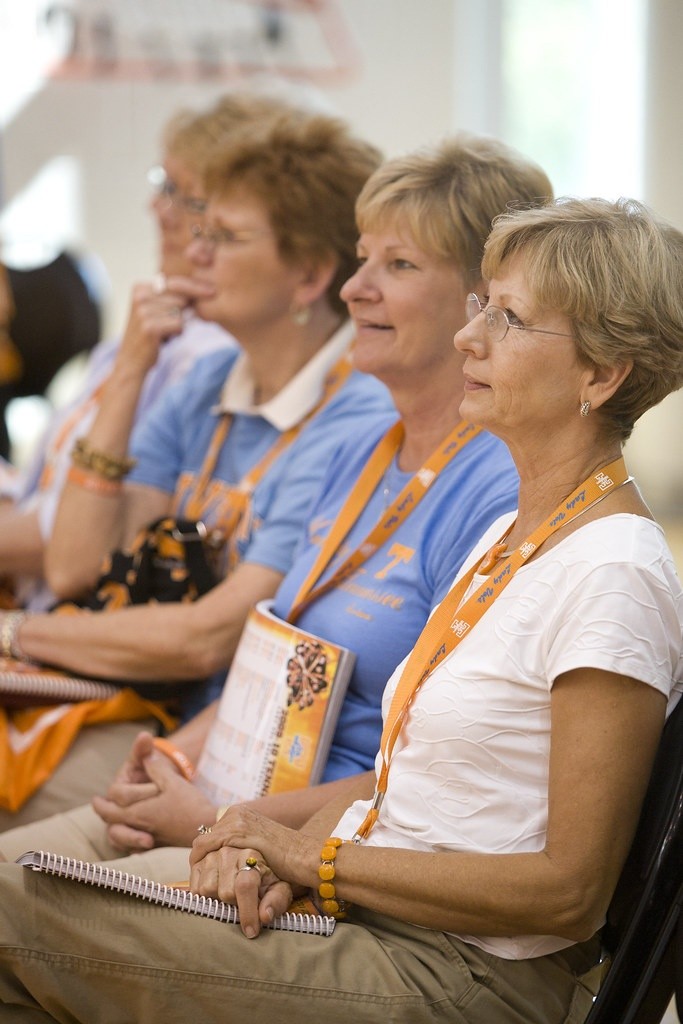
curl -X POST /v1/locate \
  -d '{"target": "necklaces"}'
[477,476,636,574]
[378,443,403,519]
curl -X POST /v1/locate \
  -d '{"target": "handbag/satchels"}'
[40,513,231,700]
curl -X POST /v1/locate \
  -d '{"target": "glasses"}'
[462,292,575,342]
[191,224,262,254]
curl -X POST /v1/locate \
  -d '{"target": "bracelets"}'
[317,837,355,917]
[64,438,135,480]
[147,737,194,784]
[2,610,23,657]
[68,467,123,493]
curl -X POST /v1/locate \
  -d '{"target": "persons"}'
[0,91,683,1024]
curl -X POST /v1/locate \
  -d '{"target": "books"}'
[14,849,335,938]
[197,599,360,820]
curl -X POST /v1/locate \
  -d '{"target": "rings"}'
[197,825,212,835]
[235,857,262,875]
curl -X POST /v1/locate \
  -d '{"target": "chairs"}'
[563,693,683,1024]
